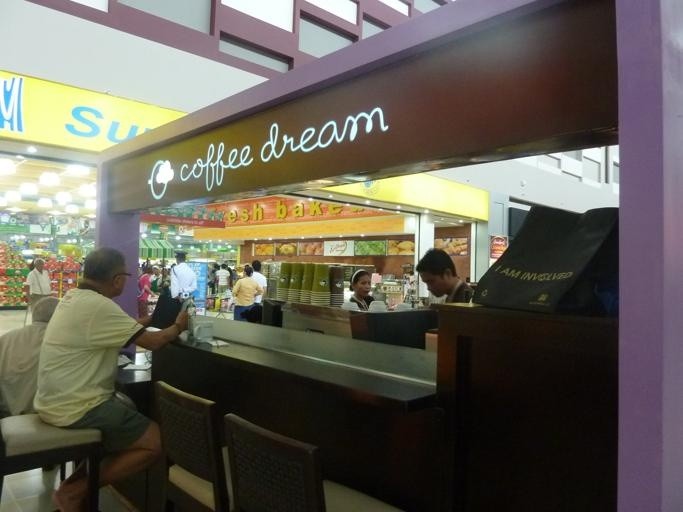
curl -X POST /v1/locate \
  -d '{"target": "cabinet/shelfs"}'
[0,257,79,310]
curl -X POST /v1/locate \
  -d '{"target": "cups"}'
[194,321,213,343]
[273,261,344,308]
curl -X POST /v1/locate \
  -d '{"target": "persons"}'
[0,295,61,420]
[349,269,374,311]
[33,248,188,512]
[139,250,196,318]
[416,248,475,303]
[25,258,51,314]
[208,260,267,323]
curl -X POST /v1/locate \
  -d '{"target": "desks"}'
[429,303,618,512]
[115,352,152,413]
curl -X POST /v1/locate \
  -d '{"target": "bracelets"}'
[174,323,181,335]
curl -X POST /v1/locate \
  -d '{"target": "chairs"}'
[222,413,408,512]
[154,380,234,512]
[0,414,103,511]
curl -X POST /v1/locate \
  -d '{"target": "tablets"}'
[150,294,187,333]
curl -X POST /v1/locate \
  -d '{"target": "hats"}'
[174,249,187,256]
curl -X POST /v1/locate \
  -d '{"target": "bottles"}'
[186,296,197,335]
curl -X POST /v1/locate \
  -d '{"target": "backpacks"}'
[137,272,147,297]
